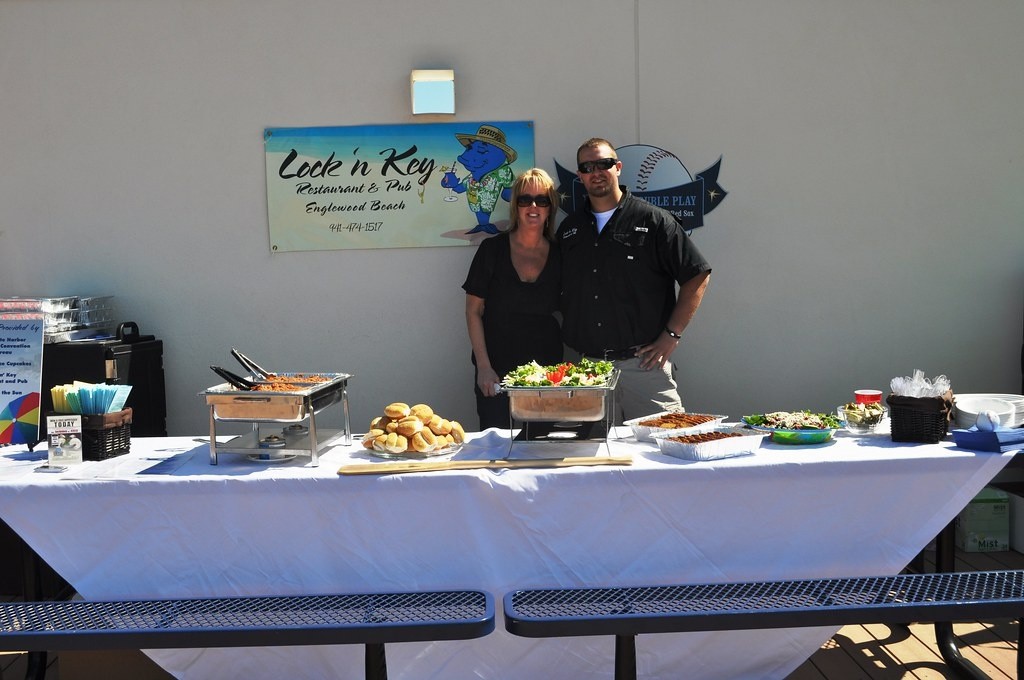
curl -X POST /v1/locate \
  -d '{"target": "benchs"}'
[502,567,1023,680]
[1,590,495,680]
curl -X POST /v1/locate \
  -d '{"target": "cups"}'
[853,388,883,404]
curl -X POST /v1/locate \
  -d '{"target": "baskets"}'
[49,410,130,461]
[886,389,951,445]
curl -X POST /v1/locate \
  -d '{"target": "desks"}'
[0,415,1024,680]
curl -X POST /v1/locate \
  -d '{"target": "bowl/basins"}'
[835,404,888,435]
[954,397,1016,428]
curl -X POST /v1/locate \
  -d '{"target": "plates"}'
[740,415,847,445]
[367,441,464,459]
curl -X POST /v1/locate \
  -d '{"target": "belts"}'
[580,344,648,362]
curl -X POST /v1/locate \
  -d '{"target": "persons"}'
[461,167,563,441]
[555,139,712,440]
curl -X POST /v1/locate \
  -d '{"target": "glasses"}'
[577,157,619,172]
[515,195,551,207]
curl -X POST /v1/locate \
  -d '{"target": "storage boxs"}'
[956,486,1011,553]
[1008,492,1024,553]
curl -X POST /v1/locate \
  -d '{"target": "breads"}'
[362,402,465,453]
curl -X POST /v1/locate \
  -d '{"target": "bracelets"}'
[664,325,681,339]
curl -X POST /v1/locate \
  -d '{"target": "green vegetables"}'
[742,409,841,431]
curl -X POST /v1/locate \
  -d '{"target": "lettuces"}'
[506,357,614,386]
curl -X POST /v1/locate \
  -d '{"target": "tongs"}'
[232,347,269,381]
[209,364,258,391]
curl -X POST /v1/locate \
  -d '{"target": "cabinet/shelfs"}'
[41,323,166,438]
